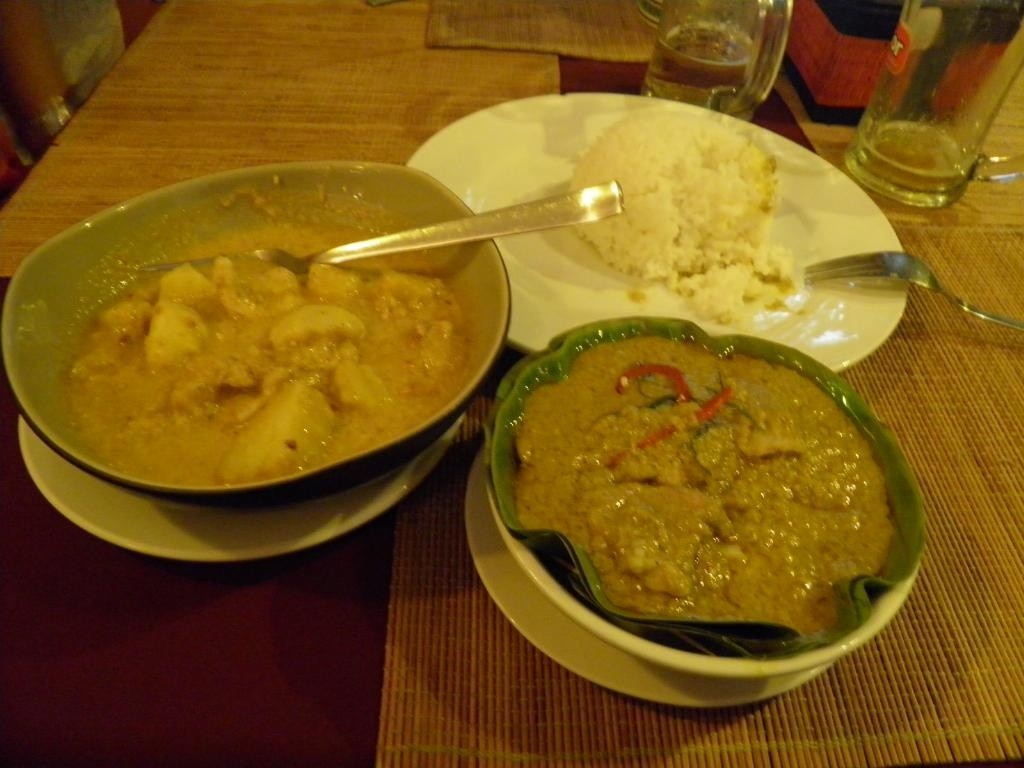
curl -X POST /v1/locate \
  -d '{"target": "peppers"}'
[607,366,733,469]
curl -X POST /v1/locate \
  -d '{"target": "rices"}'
[566,102,800,324]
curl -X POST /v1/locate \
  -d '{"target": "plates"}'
[464,443,834,707]
[18,414,466,560]
[405,91,909,373]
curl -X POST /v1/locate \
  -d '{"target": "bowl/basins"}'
[0,160,513,504]
[480,316,923,676]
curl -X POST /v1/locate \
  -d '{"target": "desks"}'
[0,1,1022,767]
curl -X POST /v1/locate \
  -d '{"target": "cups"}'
[639,0,794,121]
[844,0,1024,209]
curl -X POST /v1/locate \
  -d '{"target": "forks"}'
[139,181,625,284]
[805,251,1024,332]
